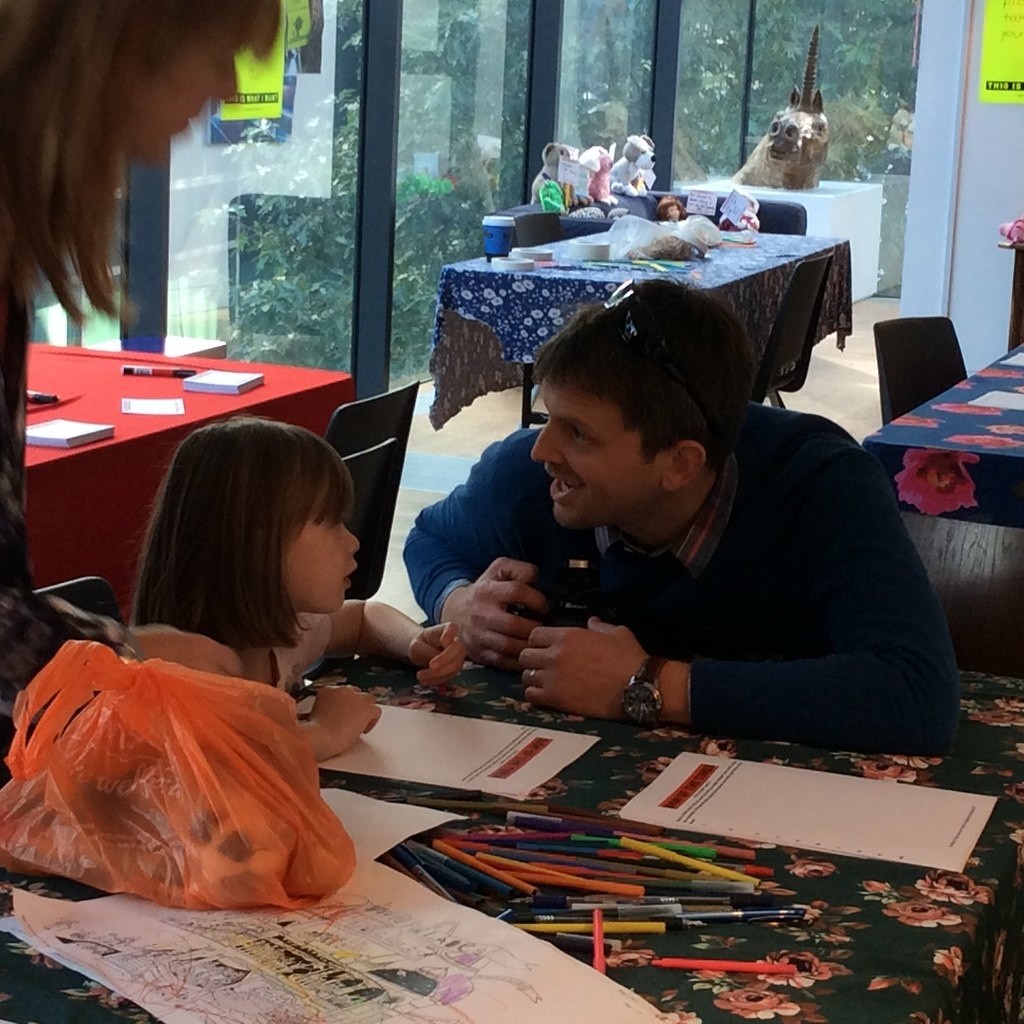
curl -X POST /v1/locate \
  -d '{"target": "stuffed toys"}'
[578,146,618,206]
[609,134,657,198]
[530,143,571,205]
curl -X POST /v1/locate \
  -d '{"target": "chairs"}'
[315,378,427,596]
[870,314,971,432]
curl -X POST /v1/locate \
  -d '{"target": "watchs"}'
[619,652,670,728]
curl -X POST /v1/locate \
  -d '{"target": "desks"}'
[18,338,354,587]
[857,339,1024,680]
[1,594,1024,1024]
[429,227,851,455]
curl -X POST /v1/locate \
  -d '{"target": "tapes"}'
[511,247,554,262]
[491,258,535,272]
[570,241,611,263]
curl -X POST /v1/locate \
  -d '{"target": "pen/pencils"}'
[380,790,807,986]
[631,259,695,273]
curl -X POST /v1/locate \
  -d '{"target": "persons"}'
[656,196,686,220]
[404,278,958,753]
[0,0,283,706]
[130,420,465,763]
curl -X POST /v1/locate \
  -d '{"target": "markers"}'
[121,365,197,378]
[25,389,60,405]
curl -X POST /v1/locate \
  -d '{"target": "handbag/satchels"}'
[0,641,357,912]
[606,213,722,260]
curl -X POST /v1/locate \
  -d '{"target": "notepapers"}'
[183,368,264,394]
[24,419,115,448]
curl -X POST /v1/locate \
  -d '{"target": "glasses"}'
[604,280,646,346]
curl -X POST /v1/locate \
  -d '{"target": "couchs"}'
[493,185,809,240]
